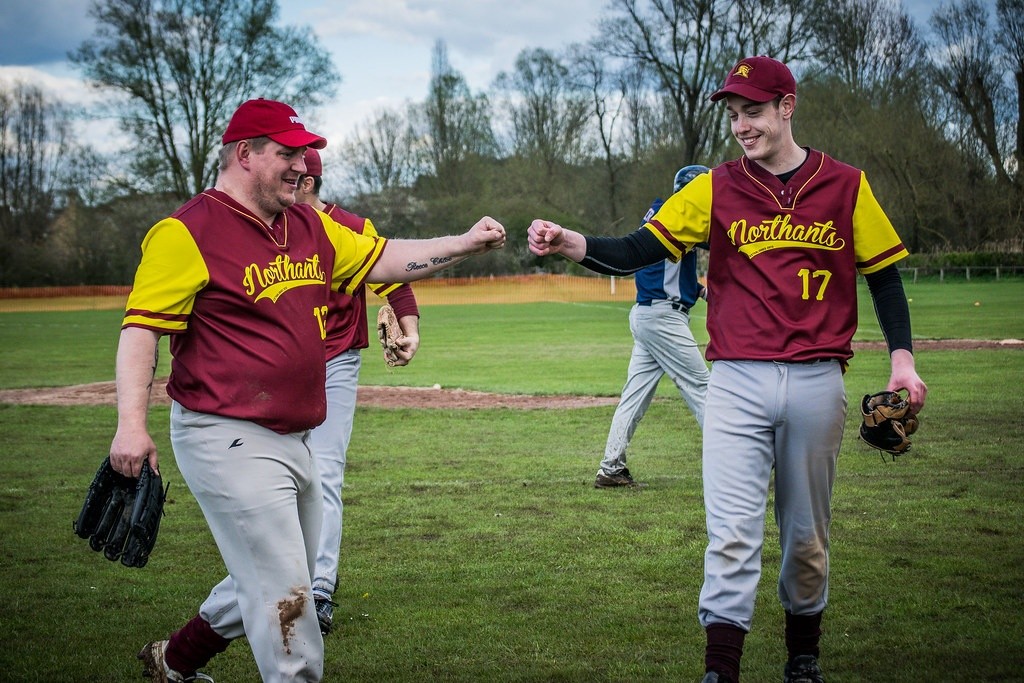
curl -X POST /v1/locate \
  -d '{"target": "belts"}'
[771,358,831,365]
[638,301,689,314]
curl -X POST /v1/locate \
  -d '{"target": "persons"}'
[593,164,709,486]
[110,96,506,682]
[295,147,420,635]
[526,55,927,683]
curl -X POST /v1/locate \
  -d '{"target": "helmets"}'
[672,164,710,191]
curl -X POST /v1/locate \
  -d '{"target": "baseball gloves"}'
[376,296,404,367]
[75,456,164,569]
[858,389,919,457]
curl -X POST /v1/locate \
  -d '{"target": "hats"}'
[222,97,327,149]
[710,54,796,101]
[304,147,322,176]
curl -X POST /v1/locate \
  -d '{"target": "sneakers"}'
[783,655,825,683]
[138,640,197,683]
[595,468,649,488]
[312,594,339,633]
[702,671,719,683]
